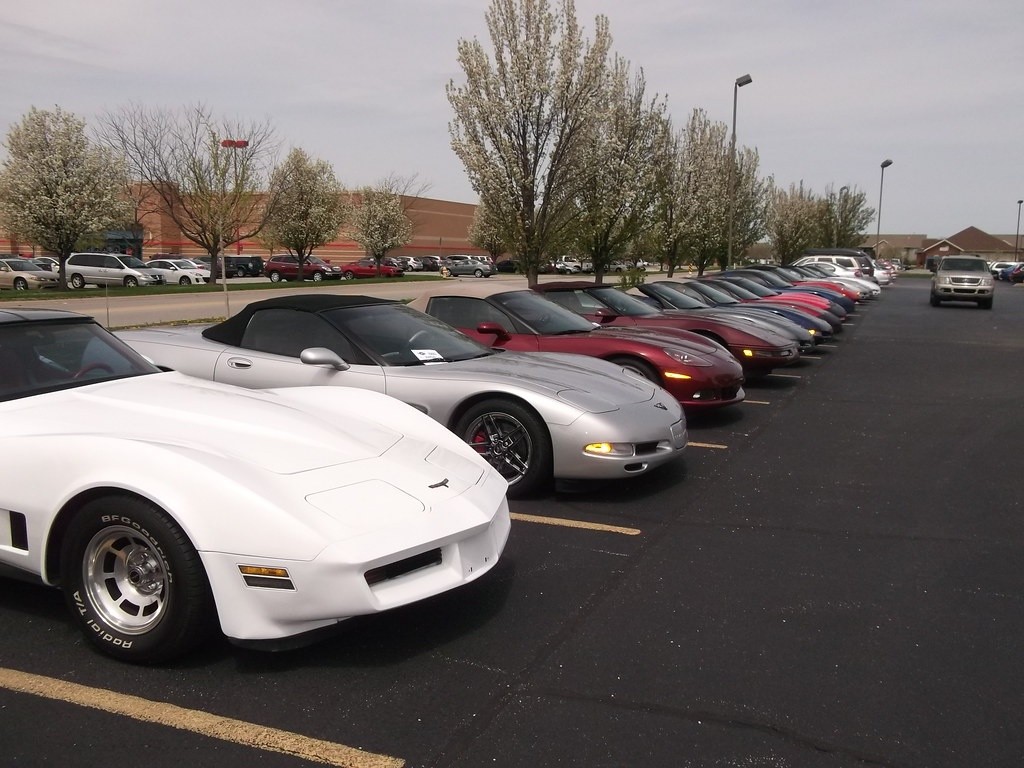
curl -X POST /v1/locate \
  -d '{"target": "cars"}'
[0,259,60,291]
[340,256,404,281]
[573,257,644,272]
[264,254,342,282]
[789,255,862,272]
[440,254,499,278]
[195,257,238,278]
[182,258,211,270]
[418,256,440,271]
[33,256,60,267]
[432,256,446,266]
[144,259,211,285]
[0,252,33,259]
[149,253,188,259]
[385,257,409,270]
[397,255,423,272]
[800,260,864,279]
[557,255,578,267]
[786,248,875,277]
[881,259,902,279]
[497,260,521,274]
[986,260,1024,283]
[554,263,581,273]
[873,263,891,286]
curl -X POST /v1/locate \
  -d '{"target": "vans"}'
[65,253,167,289]
[225,255,264,277]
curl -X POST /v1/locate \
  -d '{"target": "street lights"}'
[221,140,249,253]
[727,74,752,269]
[1015,200,1023,261]
[875,159,893,264]
[839,186,848,199]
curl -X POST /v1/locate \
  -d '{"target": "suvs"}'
[930,253,995,309]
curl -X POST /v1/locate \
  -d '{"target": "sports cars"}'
[652,266,881,345]
[79,294,689,497]
[0,307,513,667]
[620,282,817,355]
[404,283,744,412]
[531,281,800,388]
